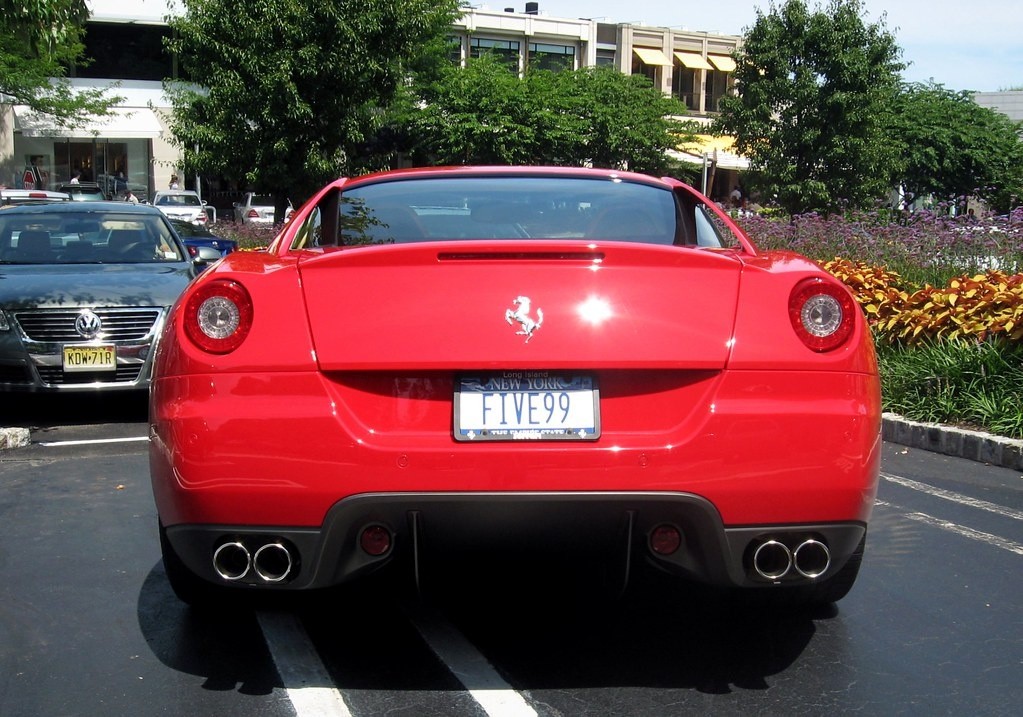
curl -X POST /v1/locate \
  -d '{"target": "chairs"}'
[16,231,51,261]
[584,204,664,240]
[108,228,143,262]
[349,203,426,243]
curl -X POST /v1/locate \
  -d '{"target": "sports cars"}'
[146,163,882,613]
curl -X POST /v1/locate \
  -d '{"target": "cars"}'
[143,190,210,226]
[233,191,295,225]
[0,200,222,395]
[140,218,241,273]
[0,173,154,252]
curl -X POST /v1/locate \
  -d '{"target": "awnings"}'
[633,48,674,66]
[12,105,163,139]
[674,52,713,70]
[708,55,737,71]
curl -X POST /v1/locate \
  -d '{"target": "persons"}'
[69,171,81,193]
[170,176,179,201]
[115,172,128,190]
[730,186,741,201]
[968,209,977,220]
[124,189,138,203]
